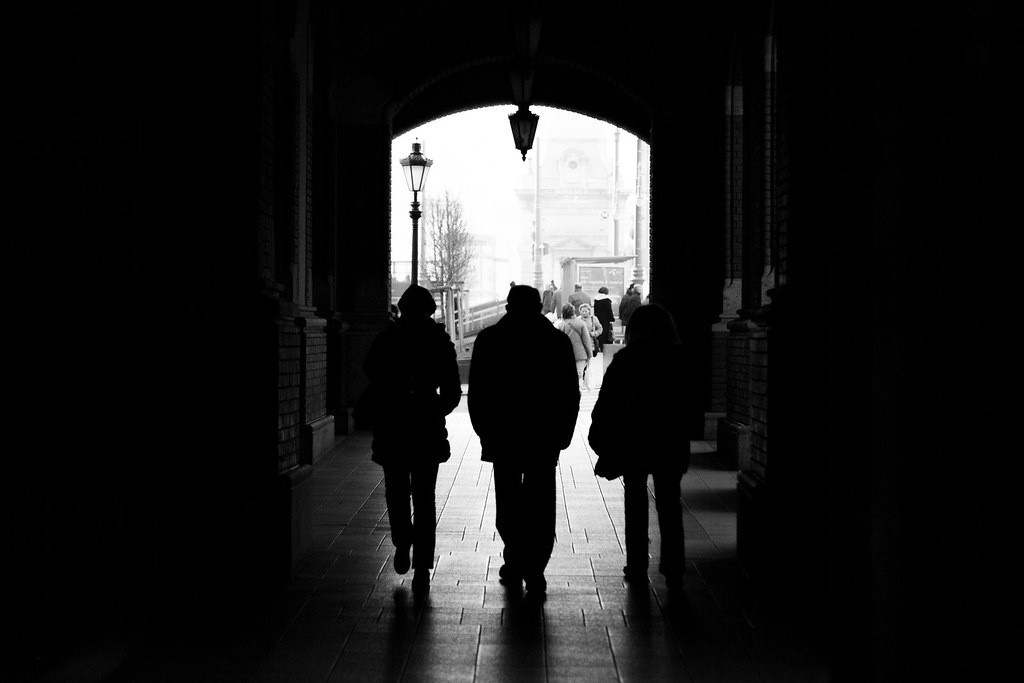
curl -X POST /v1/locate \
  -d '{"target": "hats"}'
[507,285,541,303]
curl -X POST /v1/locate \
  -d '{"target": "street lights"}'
[398,135,433,285]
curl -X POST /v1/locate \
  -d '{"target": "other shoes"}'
[415,569,430,581]
[623,566,648,584]
[659,564,683,577]
[394,543,410,574]
[499,565,522,588]
[526,581,546,591]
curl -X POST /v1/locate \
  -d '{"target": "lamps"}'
[510,78,539,162]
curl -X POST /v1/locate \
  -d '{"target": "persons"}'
[589,305,704,590]
[361,284,462,611]
[541,280,649,380]
[468,286,582,593]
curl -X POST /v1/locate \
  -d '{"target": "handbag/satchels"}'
[593,337,599,351]
[581,351,603,392]
